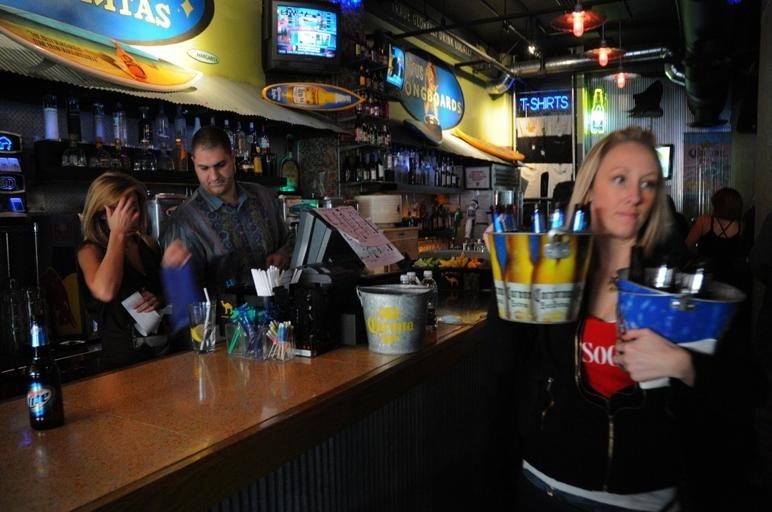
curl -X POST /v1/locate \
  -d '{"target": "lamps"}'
[551,0,605,39]
[606,47,643,87]
[581,28,622,68]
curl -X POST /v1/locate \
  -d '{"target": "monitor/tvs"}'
[365,31,407,103]
[288,209,361,268]
[264,0,342,76]
[654,143,674,181]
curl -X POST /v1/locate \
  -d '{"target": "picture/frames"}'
[464,165,492,191]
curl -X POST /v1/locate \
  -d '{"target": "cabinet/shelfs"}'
[336,140,464,254]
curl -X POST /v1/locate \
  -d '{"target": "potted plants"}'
[409,252,488,292]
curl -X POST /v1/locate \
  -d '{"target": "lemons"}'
[190,324,204,342]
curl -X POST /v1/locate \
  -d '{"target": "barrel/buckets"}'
[614,268,746,386]
[356,284,433,355]
[486,227,596,326]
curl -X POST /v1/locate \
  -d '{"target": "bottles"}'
[408,199,479,239]
[648,253,715,301]
[353,39,392,147]
[279,148,300,193]
[421,61,438,127]
[268,83,351,106]
[587,88,606,133]
[399,270,440,329]
[487,201,593,233]
[39,93,276,176]
[25,314,65,430]
[108,36,147,82]
[485,235,574,324]
[343,147,461,187]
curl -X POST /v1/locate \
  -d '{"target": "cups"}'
[188,302,217,353]
[223,323,297,362]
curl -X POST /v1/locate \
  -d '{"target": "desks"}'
[1,295,492,508]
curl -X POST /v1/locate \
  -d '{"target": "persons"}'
[67,170,163,349]
[158,124,295,310]
[684,187,753,291]
[491,127,719,512]
